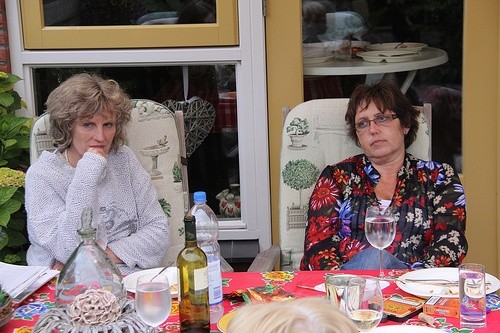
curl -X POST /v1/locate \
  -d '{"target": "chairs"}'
[30,100,185,265]
[278,99,432,272]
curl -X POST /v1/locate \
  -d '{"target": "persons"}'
[25,73,171,273]
[300,80,469,270]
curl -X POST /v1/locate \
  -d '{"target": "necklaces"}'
[65,149,69,161]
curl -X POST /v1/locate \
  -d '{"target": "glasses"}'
[350,113,399,132]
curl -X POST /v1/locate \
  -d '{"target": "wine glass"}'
[364,206,398,282]
[136,274,172,333]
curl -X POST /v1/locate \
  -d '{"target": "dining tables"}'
[301,40,448,100]
[0,265,500,333]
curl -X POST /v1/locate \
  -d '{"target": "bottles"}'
[54,227,128,308]
[176,214,210,333]
[187,191,225,325]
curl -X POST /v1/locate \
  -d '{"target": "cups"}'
[345,276,383,332]
[458,263,487,329]
[323,273,366,314]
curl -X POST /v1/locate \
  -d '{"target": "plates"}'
[365,43,428,53]
[216,307,241,333]
[356,50,422,62]
[122,267,178,295]
[314,274,390,296]
[303,40,368,50]
[303,50,336,64]
[395,268,500,300]
